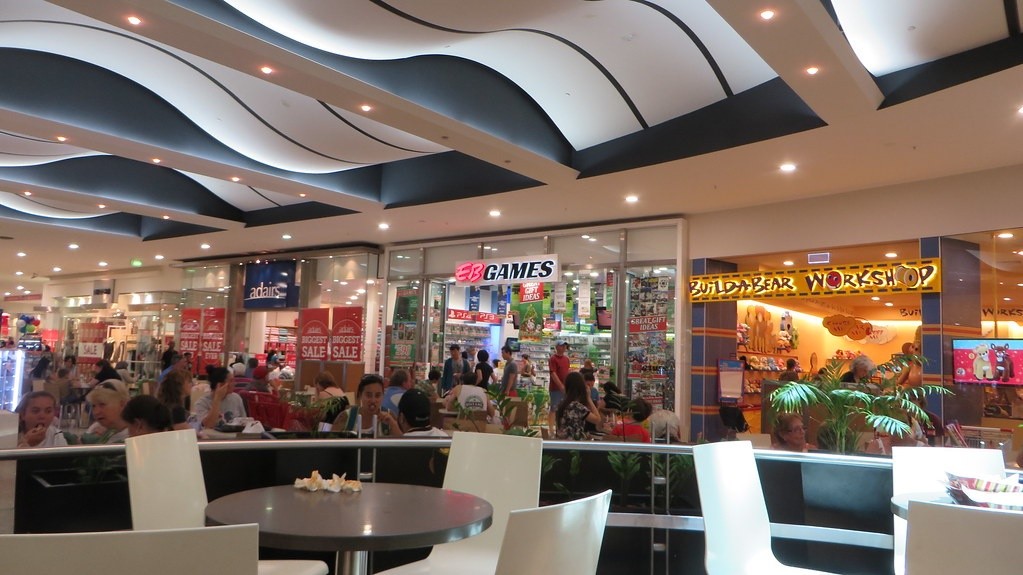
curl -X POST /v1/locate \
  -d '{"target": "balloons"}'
[16,314,40,333]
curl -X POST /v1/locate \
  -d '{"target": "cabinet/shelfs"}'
[444,323,492,363]
[265,324,297,371]
[514,334,611,390]
[736,350,799,411]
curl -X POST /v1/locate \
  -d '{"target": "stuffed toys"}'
[737,321,791,353]
[744,377,761,393]
[740,356,805,373]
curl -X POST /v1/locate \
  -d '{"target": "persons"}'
[267,349,283,369]
[29,355,52,391]
[7,337,14,348]
[910,395,947,447]
[122,394,175,437]
[57,368,81,417]
[230,354,247,377]
[647,409,817,452]
[246,358,259,379]
[442,344,532,419]
[155,355,247,440]
[65,355,84,378]
[86,379,130,443]
[14,390,69,448]
[163,340,192,370]
[315,370,448,436]
[779,355,876,382]
[85,360,122,420]
[42,339,50,352]
[548,341,651,443]
[247,366,271,392]
[115,361,140,394]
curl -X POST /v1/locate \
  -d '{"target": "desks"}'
[295,391,316,403]
[285,403,323,429]
[204,481,494,575]
[70,387,93,429]
[436,408,460,414]
[890,489,1023,520]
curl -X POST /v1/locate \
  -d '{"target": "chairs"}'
[431,400,530,432]
[0,429,1023,574]
[33,380,82,430]
[242,391,285,429]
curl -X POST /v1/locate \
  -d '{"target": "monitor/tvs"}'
[951,338,1023,387]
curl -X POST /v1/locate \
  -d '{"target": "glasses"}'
[357,374,384,398]
[780,427,808,433]
[97,381,116,392]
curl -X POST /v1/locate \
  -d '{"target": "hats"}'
[555,340,567,346]
[252,366,271,379]
[204,363,229,388]
[398,389,431,427]
